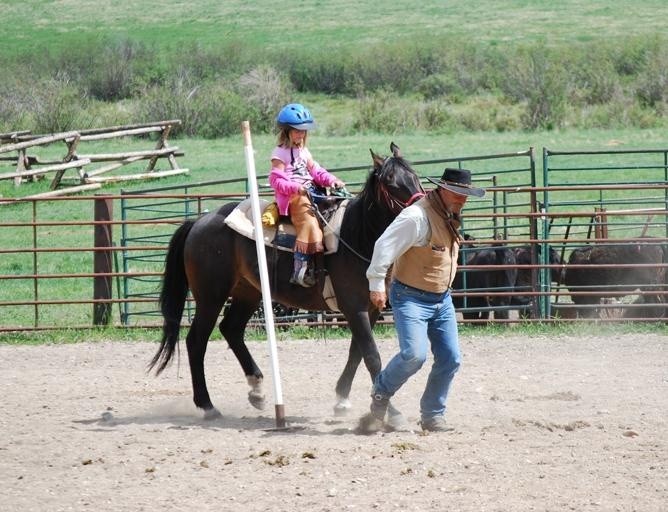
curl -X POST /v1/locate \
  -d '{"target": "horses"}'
[143,141,427,433]
[452,233,668,328]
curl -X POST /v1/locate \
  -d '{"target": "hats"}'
[426,168,486,198]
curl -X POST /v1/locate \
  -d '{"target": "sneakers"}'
[289,275,317,288]
[420,415,456,432]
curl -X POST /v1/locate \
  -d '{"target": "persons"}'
[267,102,348,290]
[356,169,486,437]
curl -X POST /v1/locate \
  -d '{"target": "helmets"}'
[277,103,316,131]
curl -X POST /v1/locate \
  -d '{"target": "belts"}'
[392,276,448,296]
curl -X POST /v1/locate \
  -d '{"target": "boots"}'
[359,389,395,433]
